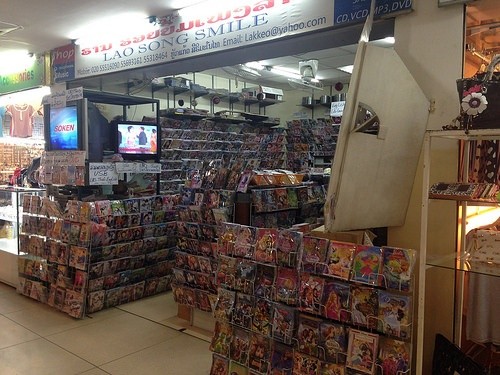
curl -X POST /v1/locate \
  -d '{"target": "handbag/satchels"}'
[456,55,500,130]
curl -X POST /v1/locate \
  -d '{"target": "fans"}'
[223,62,261,81]
[288,60,324,92]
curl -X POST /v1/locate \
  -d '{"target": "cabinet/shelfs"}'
[0,99,340,327]
[417,128,500,375]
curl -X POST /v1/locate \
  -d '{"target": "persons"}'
[126,126,135,147]
[136,126,147,153]
[150,128,157,152]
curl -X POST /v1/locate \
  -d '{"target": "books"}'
[21,196,90,320]
[171,169,328,313]
[285,117,342,168]
[160,117,285,193]
[42,151,84,186]
[209,222,413,374]
[86,194,181,313]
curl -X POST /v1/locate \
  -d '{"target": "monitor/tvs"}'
[43,98,115,163]
[114,122,161,158]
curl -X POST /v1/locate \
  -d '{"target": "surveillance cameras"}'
[302,66,313,85]
